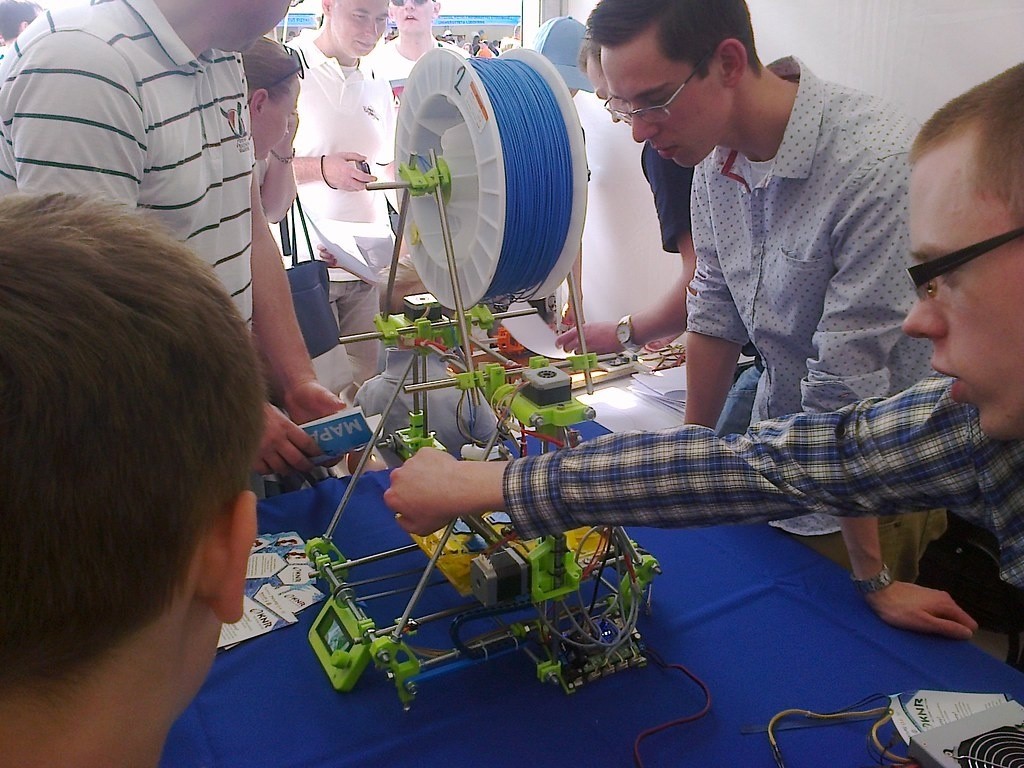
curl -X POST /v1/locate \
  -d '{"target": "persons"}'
[590,1,978,639]
[554,0,764,437]
[0,0,45,58]
[282,0,522,407]
[384,63,1024,586]
[240,37,300,224]
[0,190,265,767]
[0,0,346,477]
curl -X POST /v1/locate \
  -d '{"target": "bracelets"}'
[850,563,894,592]
[321,155,337,189]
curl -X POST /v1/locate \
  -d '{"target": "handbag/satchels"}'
[285,193,342,358]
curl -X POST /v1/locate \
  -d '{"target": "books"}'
[262,405,383,482]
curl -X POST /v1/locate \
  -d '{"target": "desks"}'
[161,354,1024,768]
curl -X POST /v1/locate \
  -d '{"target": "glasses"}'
[288,0,304,7]
[603,94,617,118]
[246,45,305,104]
[391,0,428,6]
[606,47,716,126]
[905,229,1024,303]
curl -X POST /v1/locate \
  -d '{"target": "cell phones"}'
[354,160,371,176]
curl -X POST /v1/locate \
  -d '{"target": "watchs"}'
[270,148,295,163]
[616,315,637,349]
